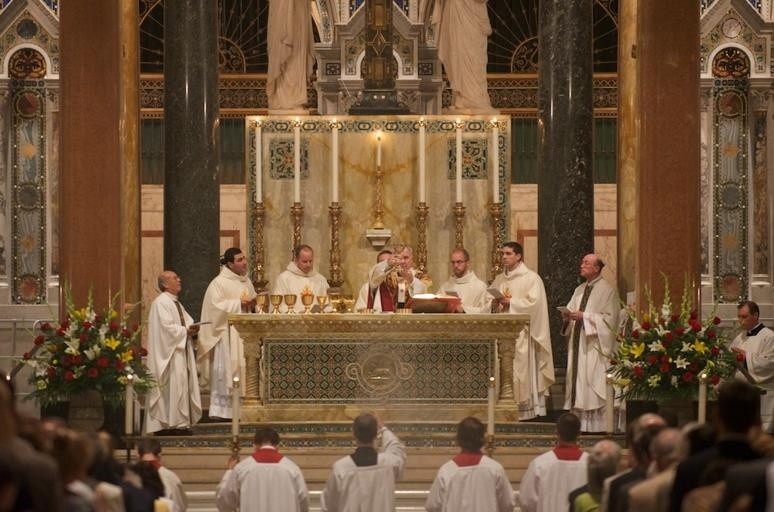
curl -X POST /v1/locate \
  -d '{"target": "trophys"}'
[254,292,328,315]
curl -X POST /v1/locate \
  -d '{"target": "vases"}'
[67,388,104,433]
[658,396,695,424]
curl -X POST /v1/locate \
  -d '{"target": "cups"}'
[502,285,512,298]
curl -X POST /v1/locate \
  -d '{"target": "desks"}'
[226,313,532,424]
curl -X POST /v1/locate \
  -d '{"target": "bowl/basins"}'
[408,295,463,312]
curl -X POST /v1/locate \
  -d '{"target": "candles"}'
[606,384,614,432]
[377,142,381,166]
[488,389,495,435]
[492,127,499,203]
[255,126,262,202]
[331,128,338,202]
[294,127,301,202]
[232,387,239,437]
[699,385,705,423]
[419,126,426,202]
[456,128,462,203]
[126,383,134,434]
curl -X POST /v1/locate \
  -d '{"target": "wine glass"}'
[256,292,356,315]
[393,240,405,273]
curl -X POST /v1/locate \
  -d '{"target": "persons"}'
[567,375,774,512]
[435,249,492,314]
[726,301,774,436]
[141,271,200,438]
[194,248,257,420]
[214,428,310,512]
[268,244,338,313]
[320,410,407,512]
[354,250,394,312]
[520,413,590,512]
[477,241,556,422]
[558,254,620,435]
[1,375,187,512]
[369,244,428,313]
[422,415,517,512]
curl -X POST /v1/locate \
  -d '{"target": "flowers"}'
[20,279,163,409]
[592,271,745,398]
